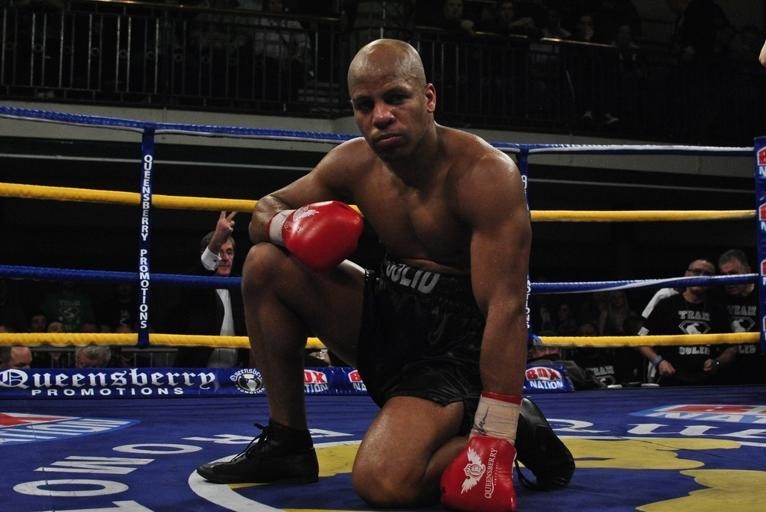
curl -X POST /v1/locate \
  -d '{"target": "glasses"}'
[688,268,713,275]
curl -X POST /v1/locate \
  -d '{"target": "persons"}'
[3,208,242,370]
[199,38,576,508]
[530,263,761,389]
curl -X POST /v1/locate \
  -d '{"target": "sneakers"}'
[197,418,318,484]
[515,396,575,490]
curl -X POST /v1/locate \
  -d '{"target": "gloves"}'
[266,200,365,272]
[439,391,524,512]
